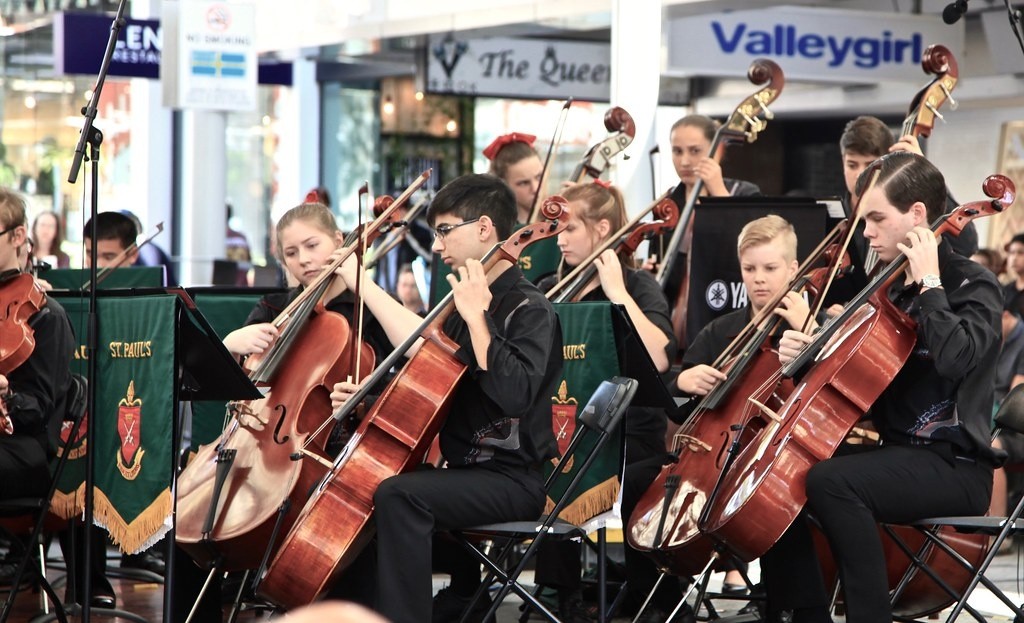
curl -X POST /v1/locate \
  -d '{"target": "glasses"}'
[432,217,496,242]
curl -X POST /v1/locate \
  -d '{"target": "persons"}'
[226,204,253,285]
[222,203,424,395]
[0,185,76,500]
[644,114,760,594]
[830,116,979,303]
[329,173,565,623]
[676,214,832,396]
[518,178,696,623]
[0,212,139,607]
[396,263,423,315]
[484,132,577,223]
[31,211,70,269]
[778,151,1005,623]
[970,232,1024,405]
[374,193,434,296]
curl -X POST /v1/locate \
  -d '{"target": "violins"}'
[0,271,49,438]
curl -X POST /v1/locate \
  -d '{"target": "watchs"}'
[918,275,943,294]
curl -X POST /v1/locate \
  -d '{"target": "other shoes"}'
[88,575,118,609]
[120,544,166,574]
[985,532,1015,554]
[429,583,497,623]
[631,598,697,623]
[556,598,592,623]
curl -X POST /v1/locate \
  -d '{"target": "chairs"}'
[440,375,640,623]
[883,381,1024,623]
[0,371,88,623]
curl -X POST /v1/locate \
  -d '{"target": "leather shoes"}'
[722,561,748,594]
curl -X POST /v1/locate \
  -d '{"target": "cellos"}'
[253,193,574,614]
[805,42,999,623]
[565,105,638,192]
[651,56,792,459]
[661,172,1019,623]
[427,194,683,471]
[625,237,850,622]
[173,190,407,623]
